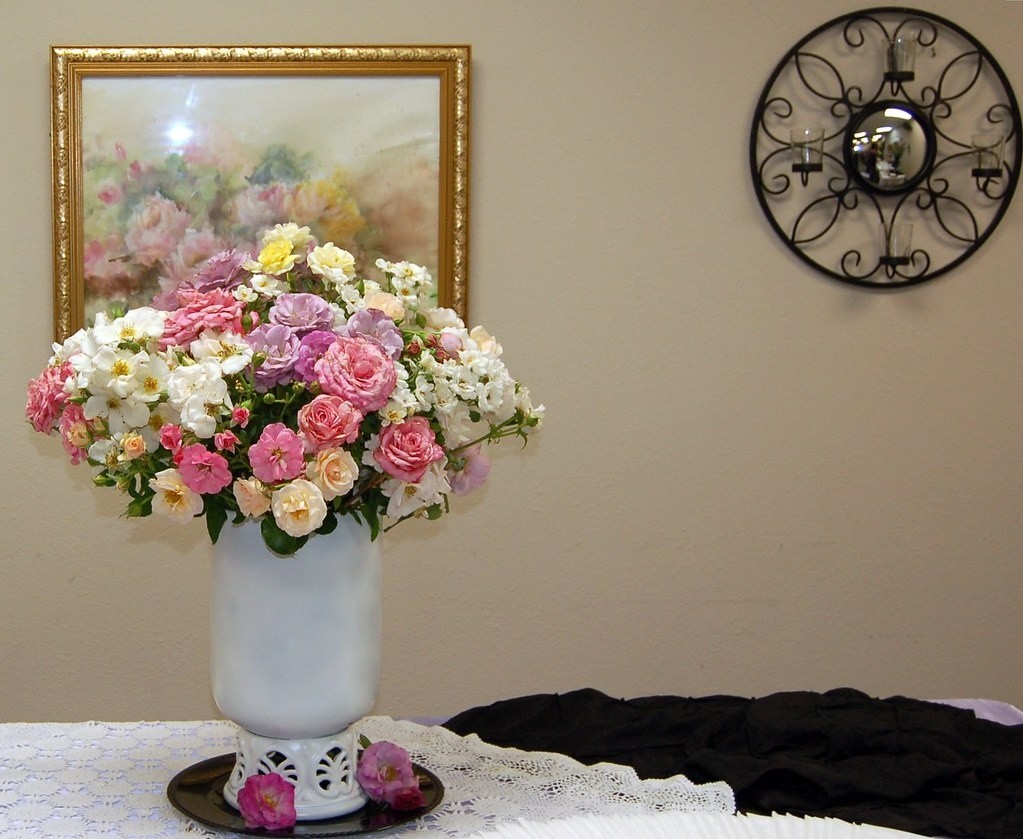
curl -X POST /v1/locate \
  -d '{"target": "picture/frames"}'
[48,42,473,359]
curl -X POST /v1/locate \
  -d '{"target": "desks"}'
[0,687,1023,839]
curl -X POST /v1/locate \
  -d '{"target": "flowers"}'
[237,769,297,830]
[23,221,546,553]
[352,731,431,810]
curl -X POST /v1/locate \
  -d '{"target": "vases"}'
[206,497,388,820]
